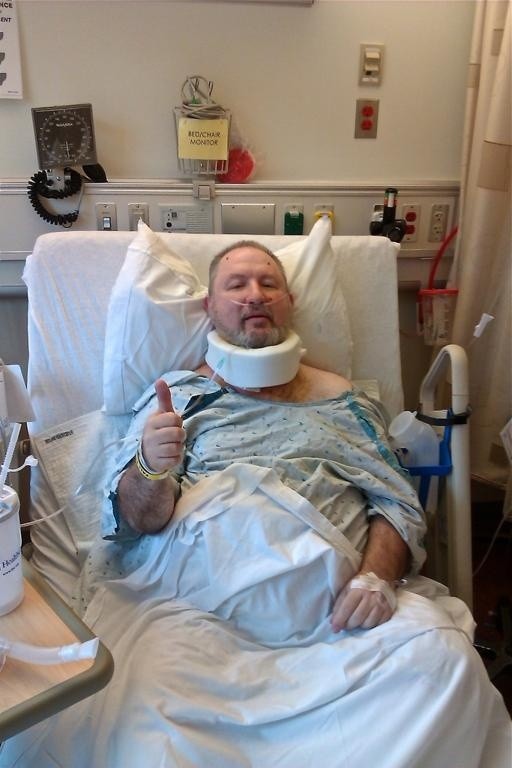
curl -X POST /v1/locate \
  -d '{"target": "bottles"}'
[0,484,24,616]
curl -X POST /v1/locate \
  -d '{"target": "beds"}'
[0,231,512,768]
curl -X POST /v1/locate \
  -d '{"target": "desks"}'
[0,551,115,745]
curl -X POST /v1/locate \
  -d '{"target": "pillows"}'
[102,216,355,416]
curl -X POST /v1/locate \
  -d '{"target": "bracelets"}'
[134,443,168,480]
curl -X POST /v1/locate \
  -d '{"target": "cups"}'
[420,288,460,347]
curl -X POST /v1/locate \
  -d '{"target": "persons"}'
[108,241,427,634]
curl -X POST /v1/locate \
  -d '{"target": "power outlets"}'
[128,202,149,231]
[314,204,335,235]
[428,203,449,243]
[402,203,420,243]
